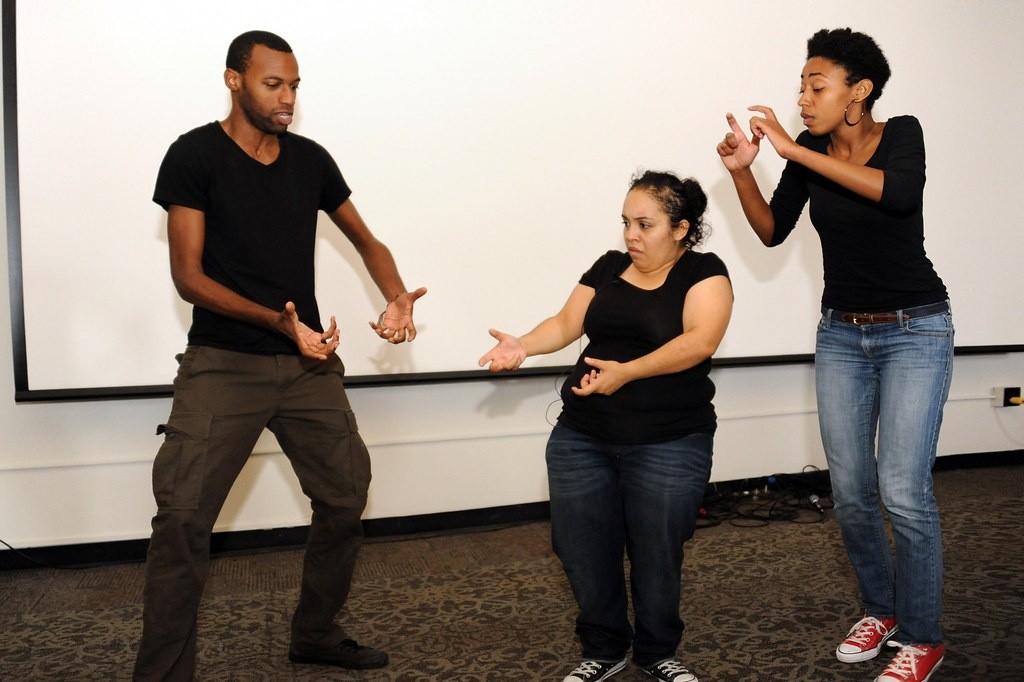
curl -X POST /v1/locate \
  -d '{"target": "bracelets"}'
[387,294,400,307]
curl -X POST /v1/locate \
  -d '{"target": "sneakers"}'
[835,614,899,664]
[637,658,698,682]
[289,639,389,670]
[563,656,628,682]
[871,641,944,682]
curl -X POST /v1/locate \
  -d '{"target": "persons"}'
[131,30,427,682]
[478,169,736,682]
[717,27,956,682]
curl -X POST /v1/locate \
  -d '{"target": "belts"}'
[820,301,948,325]
